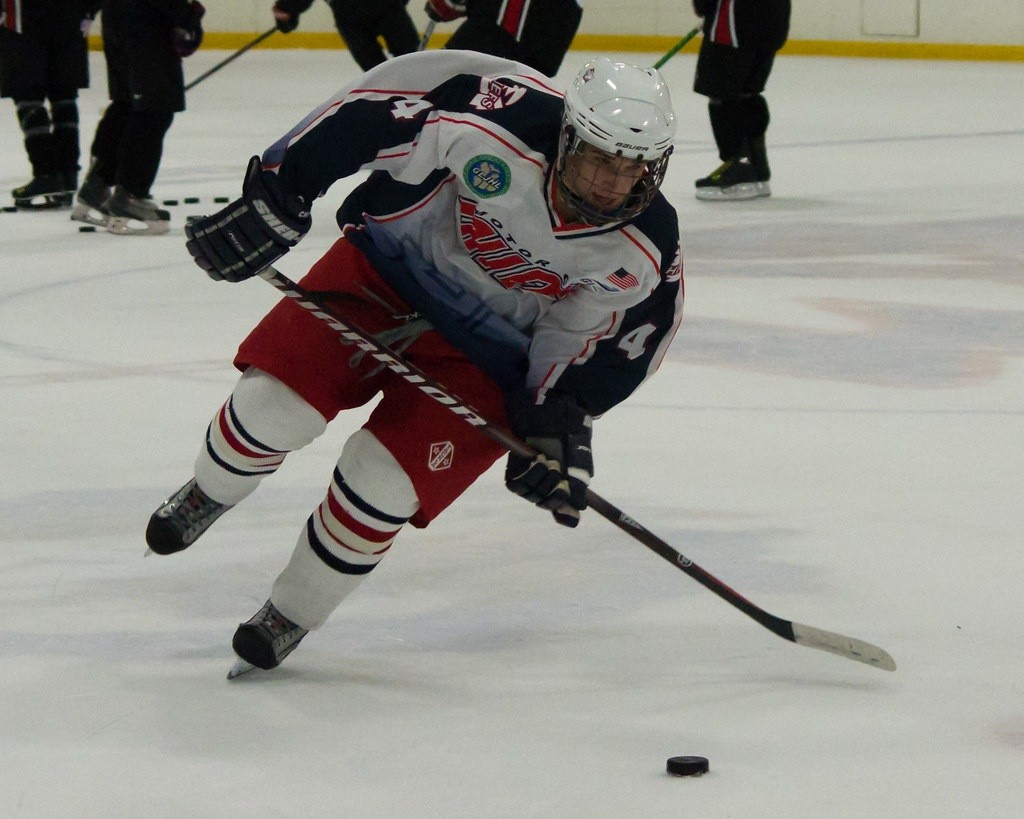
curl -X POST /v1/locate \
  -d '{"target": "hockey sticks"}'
[256,265,895,675]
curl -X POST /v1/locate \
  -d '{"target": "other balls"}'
[666,756,711,776]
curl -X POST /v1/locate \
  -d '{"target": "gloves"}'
[270,3,298,33]
[504,404,593,528]
[179,0,206,55]
[186,154,311,283]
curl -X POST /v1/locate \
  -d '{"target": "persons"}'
[422,0,583,79]
[689,0,793,201]
[70,0,207,237]
[143,49,686,684]
[0,0,91,215]
[271,0,422,73]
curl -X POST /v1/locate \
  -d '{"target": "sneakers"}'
[71,172,114,226]
[226,597,309,679]
[145,477,234,554]
[9,177,73,209]
[105,184,172,234]
[692,160,772,200]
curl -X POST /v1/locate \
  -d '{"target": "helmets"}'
[555,56,677,223]
